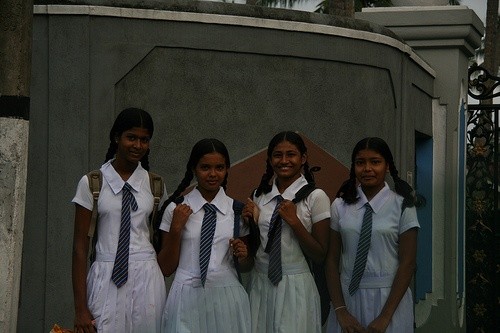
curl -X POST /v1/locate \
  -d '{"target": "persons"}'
[321,137,426,333]
[241,131,332,333]
[72,108,167,333]
[157,138,254,333]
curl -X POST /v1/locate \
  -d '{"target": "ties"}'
[349,203,373,296]
[111,183,138,288]
[267,196,283,287]
[199,203,217,288]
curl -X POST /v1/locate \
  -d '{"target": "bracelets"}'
[335,305,347,311]
